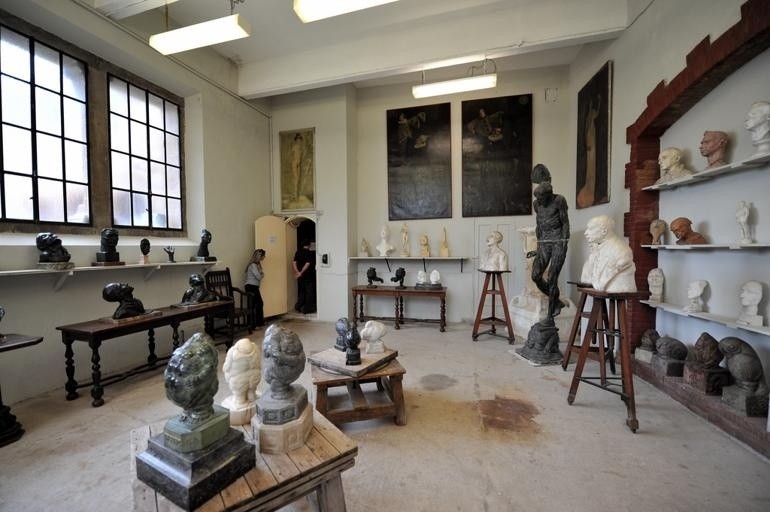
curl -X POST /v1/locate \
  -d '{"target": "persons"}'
[292,235,314,314]
[645,266,765,328]
[289,132,305,201]
[577,93,601,206]
[182,273,217,304]
[524,182,569,327]
[245,247,265,333]
[645,103,770,187]
[95,227,121,255]
[479,232,509,273]
[650,198,754,247]
[198,229,212,257]
[579,214,637,294]
[35,231,65,257]
[103,282,146,317]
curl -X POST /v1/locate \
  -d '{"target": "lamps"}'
[201,267,261,343]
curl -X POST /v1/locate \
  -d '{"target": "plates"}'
[636,145,769,338]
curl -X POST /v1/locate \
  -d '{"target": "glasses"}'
[290,0,399,29]
[148,0,261,58]
[404,54,501,102]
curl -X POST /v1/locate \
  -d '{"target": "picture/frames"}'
[55,299,234,408]
[349,277,451,333]
[304,344,415,428]
[127,400,362,512]
[1,329,47,448]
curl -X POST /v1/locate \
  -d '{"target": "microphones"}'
[563,286,656,432]
[561,275,617,378]
[470,265,517,345]
